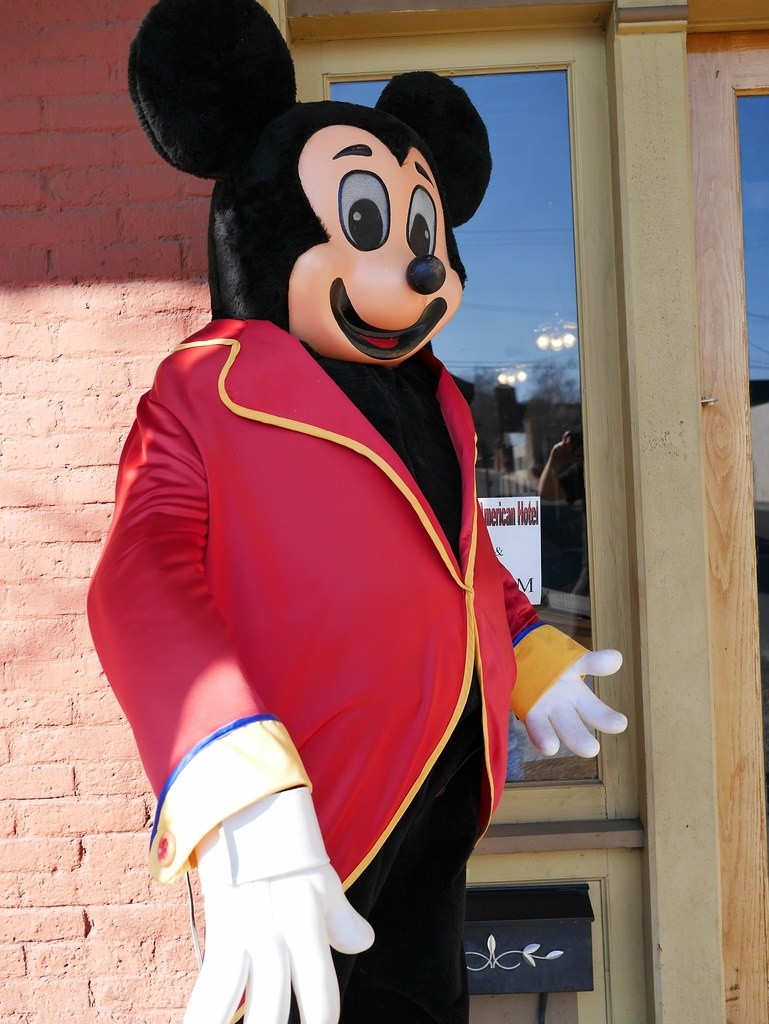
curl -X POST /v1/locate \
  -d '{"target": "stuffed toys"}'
[85,0,629,1024]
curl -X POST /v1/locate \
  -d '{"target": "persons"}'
[534,419,589,642]
